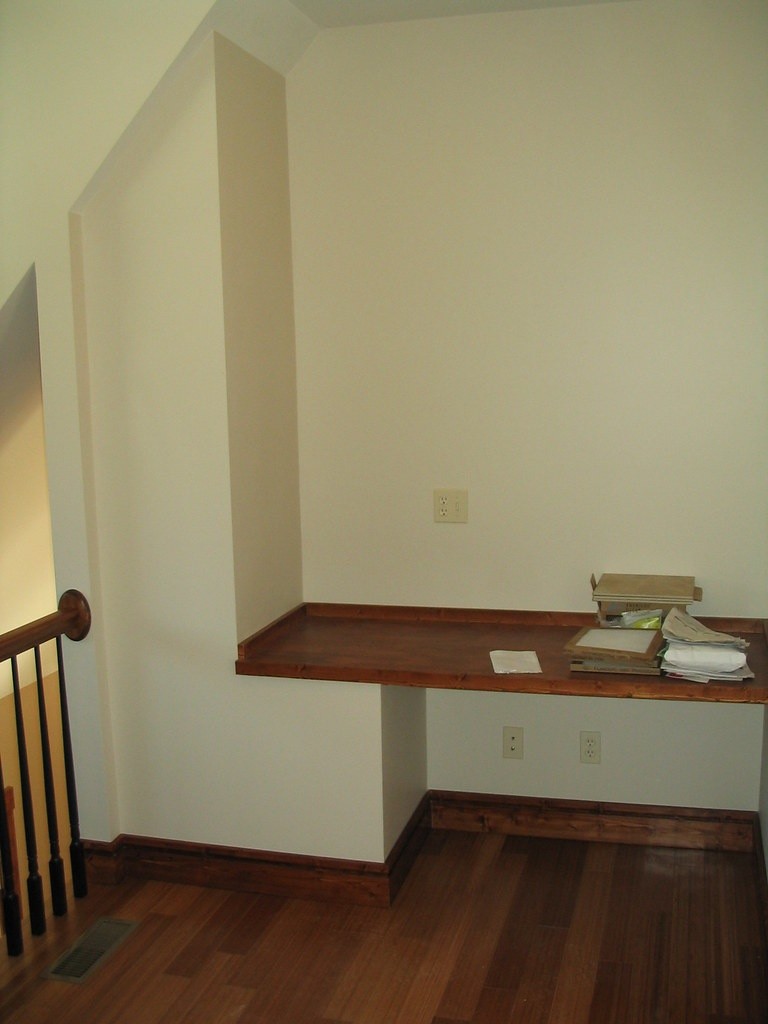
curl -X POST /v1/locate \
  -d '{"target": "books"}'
[592,572,695,606]
[562,627,755,685]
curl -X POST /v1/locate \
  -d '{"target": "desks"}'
[236,602,768,705]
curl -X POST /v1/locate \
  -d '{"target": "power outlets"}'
[581,730,601,764]
[434,490,467,523]
